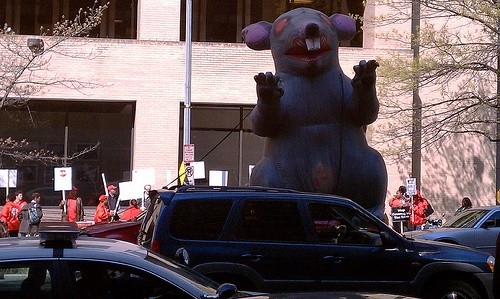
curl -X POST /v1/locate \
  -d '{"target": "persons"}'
[59,185,84,222]
[457,198,472,213]
[497,189,500,205]
[95,184,151,224]
[0,190,43,236]
[390,186,427,234]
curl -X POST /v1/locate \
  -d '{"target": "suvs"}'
[136,183,494,299]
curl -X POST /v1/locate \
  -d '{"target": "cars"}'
[401,205,500,289]
[79,207,150,245]
[0,219,421,299]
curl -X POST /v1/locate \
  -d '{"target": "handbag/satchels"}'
[423,198,434,216]
[28,203,43,224]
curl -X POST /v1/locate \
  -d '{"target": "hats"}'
[99,195,109,201]
[108,185,117,191]
[72,186,78,192]
